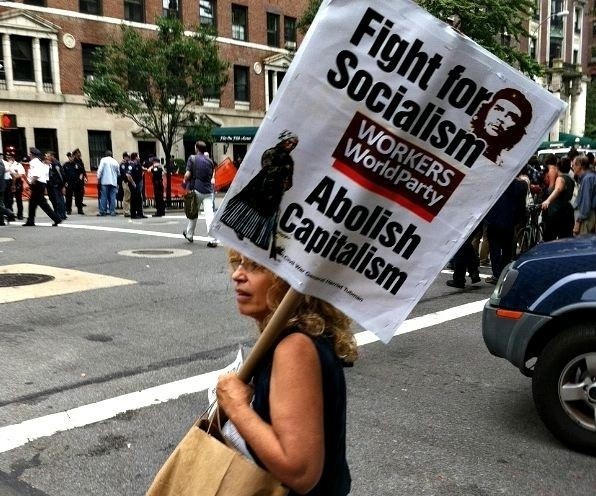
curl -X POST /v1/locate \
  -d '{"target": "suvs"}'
[482,234,596,456]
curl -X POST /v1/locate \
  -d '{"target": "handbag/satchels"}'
[143,396,290,495]
[183,190,199,220]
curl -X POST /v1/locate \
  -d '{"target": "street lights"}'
[575,135,581,149]
[526,9,570,59]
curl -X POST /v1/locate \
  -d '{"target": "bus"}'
[537,147,596,170]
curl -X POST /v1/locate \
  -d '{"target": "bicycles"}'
[512,204,546,262]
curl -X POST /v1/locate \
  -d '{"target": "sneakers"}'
[183,231,194,243]
[445,258,497,287]
[0,201,87,220]
[52,218,63,226]
[22,222,36,226]
[207,242,218,248]
[97,210,166,219]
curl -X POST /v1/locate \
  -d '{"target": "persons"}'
[220,130,299,250]
[468,88,533,166]
[216,248,357,496]
[0,140,218,247]
[446,150,596,288]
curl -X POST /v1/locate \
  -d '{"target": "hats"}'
[30,147,42,157]
[278,128,299,142]
[493,87,533,121]
[72,148,79,155]
[151,157,160,162]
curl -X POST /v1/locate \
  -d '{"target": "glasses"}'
[230,258,267,273]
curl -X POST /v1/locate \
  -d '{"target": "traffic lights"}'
[1,113,18,130]
